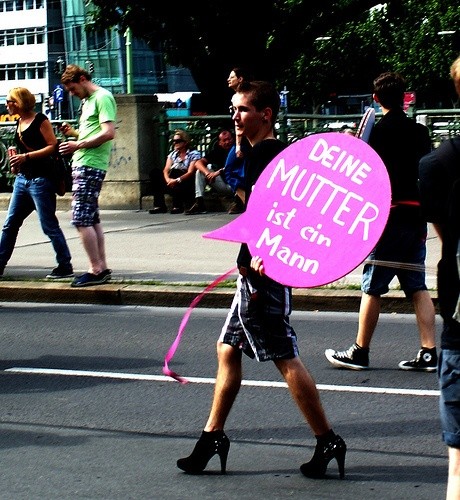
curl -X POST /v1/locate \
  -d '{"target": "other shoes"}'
[170,205,185,214]
[149,204,168,214]
[228,202,244,214]
[185,203,205,214]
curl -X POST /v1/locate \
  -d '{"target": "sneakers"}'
[70,272,106,288]
[325,343,370,370]
[399,347,438,372]
[46,265,74,278]
[105,269,113,280]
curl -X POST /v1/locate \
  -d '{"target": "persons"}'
[224,67,253,204]
[148,129,245,215]
[0,86,74,279]
[176,82,347,481]
[59,64,117,287]
[416,55,460,500]
[323,71,438,372]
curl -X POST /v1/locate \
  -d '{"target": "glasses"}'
[172,138,185,144]
[6,100,15,104]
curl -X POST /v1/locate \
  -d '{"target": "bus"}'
[154,91,208,130]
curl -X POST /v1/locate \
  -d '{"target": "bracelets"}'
[25,153,29,159]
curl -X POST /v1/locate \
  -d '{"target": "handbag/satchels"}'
[51,157,73,196]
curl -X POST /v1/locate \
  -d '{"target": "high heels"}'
[177,431,230,474]
[301,435,347,479]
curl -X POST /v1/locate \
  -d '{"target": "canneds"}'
[8,146,19,173]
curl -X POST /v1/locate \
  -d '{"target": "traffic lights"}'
[60,60,67,74]
[45,97,50,107]
[54,60,61,75]
[86,60,95,74]
